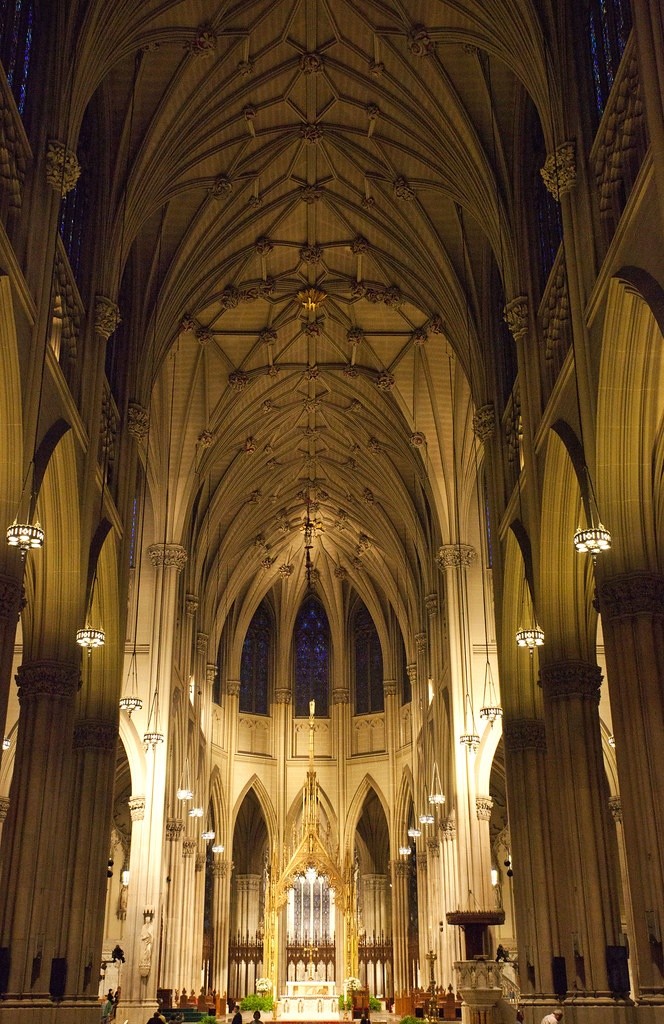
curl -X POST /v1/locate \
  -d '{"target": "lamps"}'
[144,353,178,752]
[419,814,435,830]
[508,366,547,660]
[447,354,481,754]
[428,760,447,805]
[212,845,225,859]
[399,846,411,861]
[175,471,202,806]
[117,228,164,717]
[457,204,505,729]
[75,45,142,658]
[4,0,83,563]
[202,829,215,844]
[189,805,204,823]
[407,825,422,842]
[551,0,613,564]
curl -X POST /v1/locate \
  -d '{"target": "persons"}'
[232,1006,242,1024]
[100,986,122,1024]
[249,1011,262,1024]
[147,1009,182,1024]
[541,1010,564,1024]
[139,906,153,977]
[496,944,507,962]
